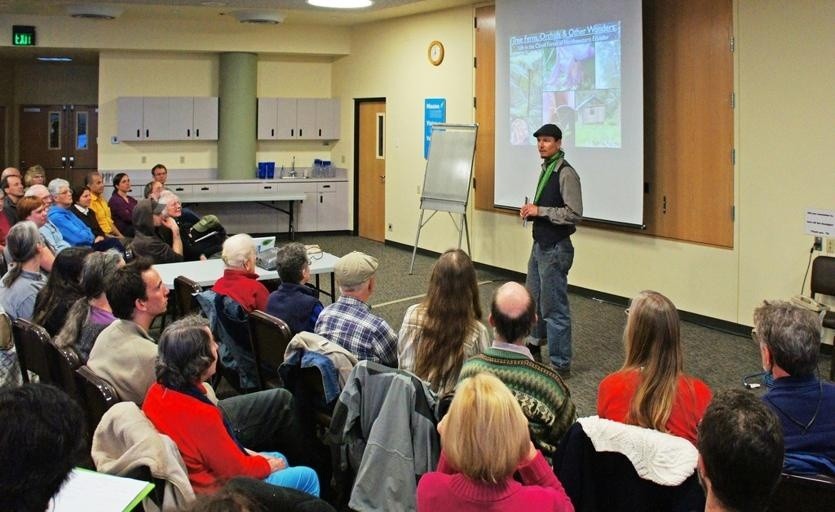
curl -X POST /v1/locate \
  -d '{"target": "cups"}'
[260,161,275,179]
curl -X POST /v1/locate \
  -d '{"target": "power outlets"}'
[815,237,823,251]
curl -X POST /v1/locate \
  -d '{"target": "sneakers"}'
[554,369,570,379]
[527,344,542,362]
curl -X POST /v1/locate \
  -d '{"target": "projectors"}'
[255,247,279,270]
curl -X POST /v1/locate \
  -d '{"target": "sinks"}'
[282,176,306,180]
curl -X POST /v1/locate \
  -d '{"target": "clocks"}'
[428,41,445,66]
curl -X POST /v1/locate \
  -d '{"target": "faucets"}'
[292,157,295,171]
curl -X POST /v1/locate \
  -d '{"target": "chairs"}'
[810,255,835,359]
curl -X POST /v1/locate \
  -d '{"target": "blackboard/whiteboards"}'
[420,124,478,213]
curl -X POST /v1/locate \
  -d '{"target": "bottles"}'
[281,159,332,179]
[125,241,134,263]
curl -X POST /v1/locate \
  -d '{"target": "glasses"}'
[58,190,73,194]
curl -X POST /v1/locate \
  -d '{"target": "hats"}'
[533,124,562,136]
[334,251,378,284]
[152,200,167,214]
[193,215,219,232]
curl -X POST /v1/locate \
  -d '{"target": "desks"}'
[148,251,340,334]
[173,190,306,242]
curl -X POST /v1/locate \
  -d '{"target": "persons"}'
[519,123,584,379]
[1,165,834,511]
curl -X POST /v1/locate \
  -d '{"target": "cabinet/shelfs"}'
[101,179,350,235]
[257,97,341,141]
[115,96,218,142]
